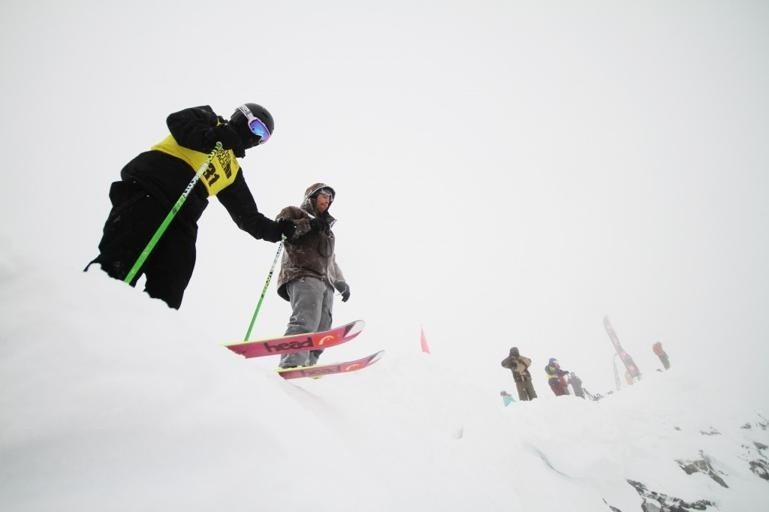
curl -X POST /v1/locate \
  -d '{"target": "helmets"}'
[509,347,519,356]
[231,103,275,149]
[549,358,557,366]
[304,182,334,200]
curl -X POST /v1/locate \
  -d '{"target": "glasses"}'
[320,187,334,202]
[237,103,271,145]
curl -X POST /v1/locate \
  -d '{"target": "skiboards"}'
[226,318,381,380]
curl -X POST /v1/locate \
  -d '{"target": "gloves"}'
[334,282,350,302]
[203,123,245,158]
[276,217,296,240]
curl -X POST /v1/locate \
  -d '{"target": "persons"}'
[546,355,588,399]
[502,346,538,400]
[274,183,351,369]
[501,391,514,405]
[83,102,273,308]
